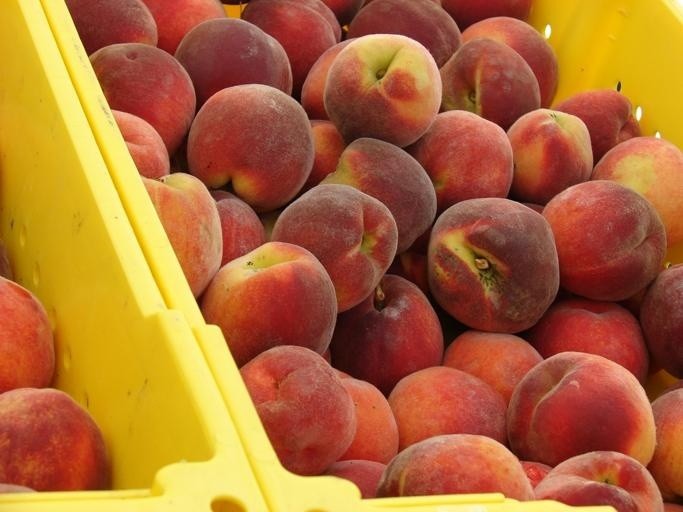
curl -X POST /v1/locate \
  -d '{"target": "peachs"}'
[0,272,56,391]
[1,388,110,492]
[68,1,683,512]
